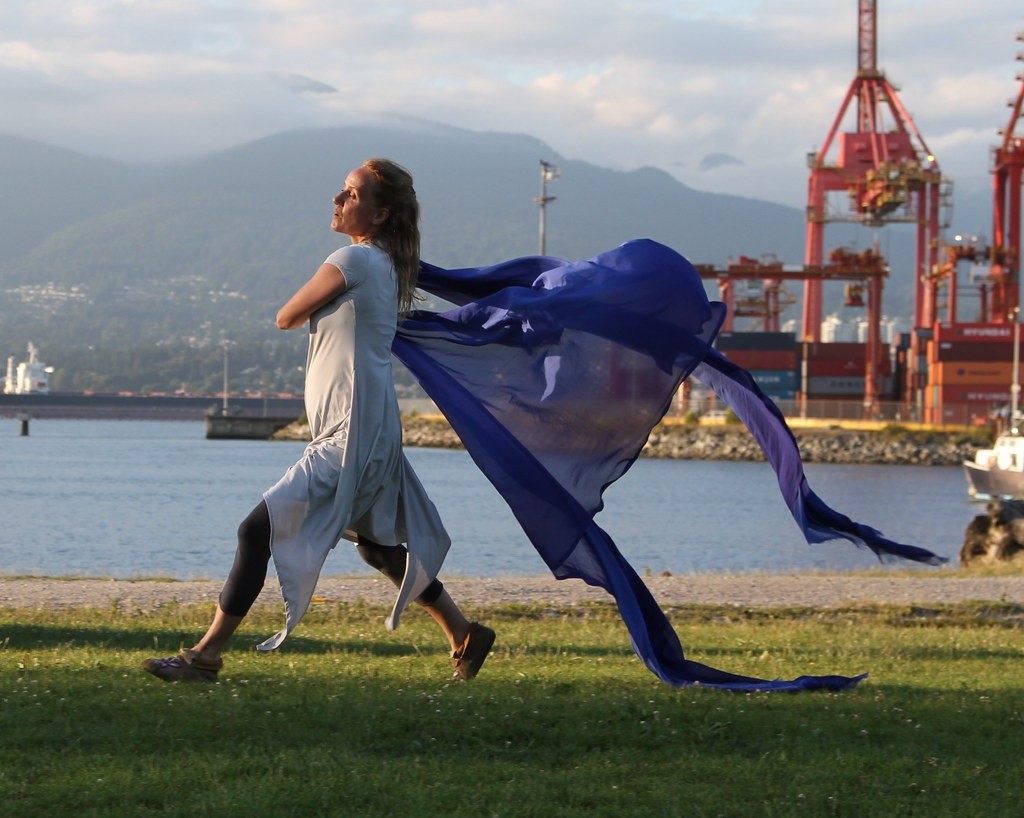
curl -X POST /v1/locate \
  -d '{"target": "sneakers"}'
[141,648,222,684]
[450,621,495,679]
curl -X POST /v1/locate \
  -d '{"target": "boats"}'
[965,305,1022,505]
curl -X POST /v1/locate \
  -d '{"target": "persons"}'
[141,159,499,690]
